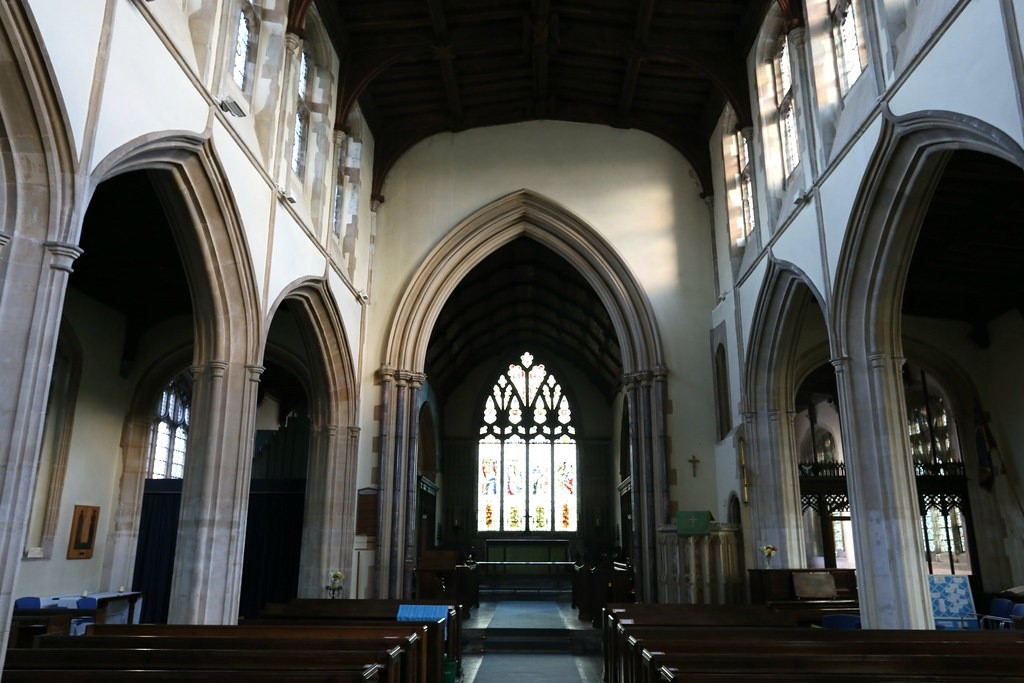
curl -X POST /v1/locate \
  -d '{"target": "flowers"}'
[760,544,777,558]
[328,571,345,580]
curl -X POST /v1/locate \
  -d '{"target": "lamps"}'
[221,90,249,116]
[792,189,805,204]
[718,290,727,301]
[357,287,368,301]
[284,188,296,204]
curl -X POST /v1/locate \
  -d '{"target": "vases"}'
[332,578,339,586]
[766,555,772,569]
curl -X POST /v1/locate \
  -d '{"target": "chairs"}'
[978,597,1024,629]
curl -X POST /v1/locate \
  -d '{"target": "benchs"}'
[1,602,463,683]
[598,599,1024,683]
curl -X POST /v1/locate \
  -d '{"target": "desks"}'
[43,591,142,634]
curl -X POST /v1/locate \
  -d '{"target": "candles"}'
[118,586,125,591]
[81,590,87,596]
